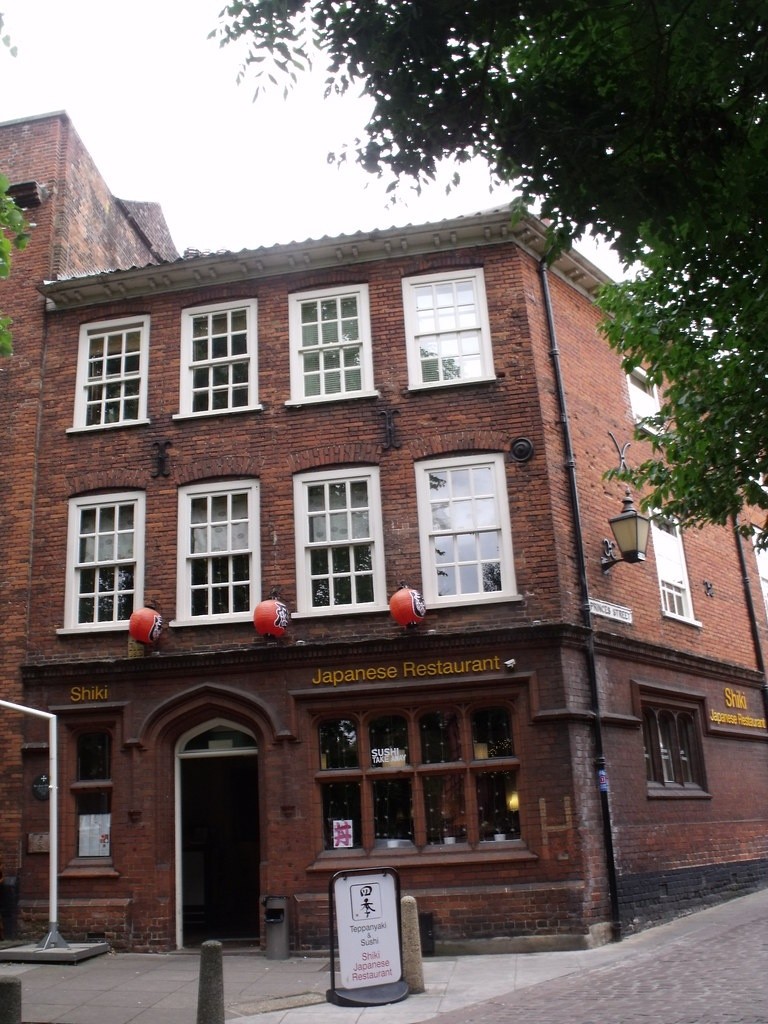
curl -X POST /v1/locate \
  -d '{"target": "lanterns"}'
[388,588,425,626]
[129,608,162,644]
[254,600,291,635]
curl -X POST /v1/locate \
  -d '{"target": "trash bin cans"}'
[262,895,290,960]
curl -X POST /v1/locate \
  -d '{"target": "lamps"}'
[503,783,522,813]
[473,739,490,763]
[601,486,653,569]
